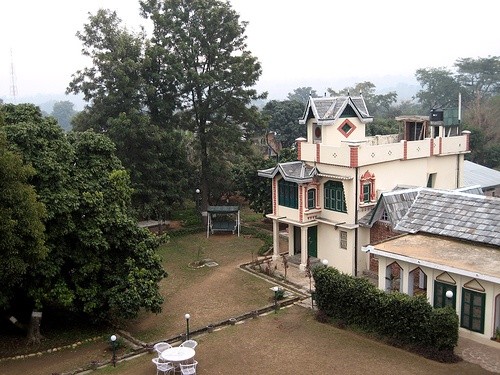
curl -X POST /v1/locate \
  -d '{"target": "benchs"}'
[210,221,238,234]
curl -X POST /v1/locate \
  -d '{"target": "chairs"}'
[179,339,199,363]
[154,342,174,366]
[179,360,198,375]
[152,357,175,375]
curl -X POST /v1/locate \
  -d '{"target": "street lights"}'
[195,189,201,212]
[446,290,453,306]
[185,314,191,340]
[323,259,328,268]
[111,335,116,368]
[274,287,278,313]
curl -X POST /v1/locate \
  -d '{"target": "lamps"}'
[334,222,346,230]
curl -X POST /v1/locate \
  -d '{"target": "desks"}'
[161,347,195,375]
